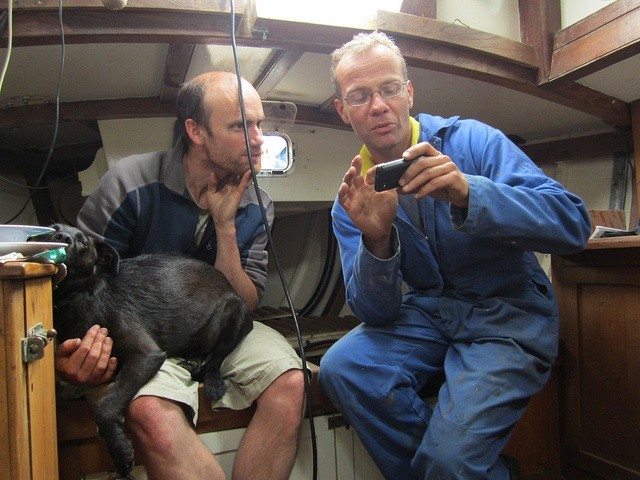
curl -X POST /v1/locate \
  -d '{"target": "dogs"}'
[28,219,254,475]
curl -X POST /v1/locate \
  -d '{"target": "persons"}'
[316,28,591,479]
[52,70,313,480]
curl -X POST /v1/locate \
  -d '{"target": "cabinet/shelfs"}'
[66,354,507,480]
[0,263,59,480]
[552,242,640,480]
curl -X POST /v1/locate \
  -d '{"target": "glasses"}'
[337,81,408,106]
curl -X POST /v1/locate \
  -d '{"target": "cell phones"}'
[376,159,411,189]
